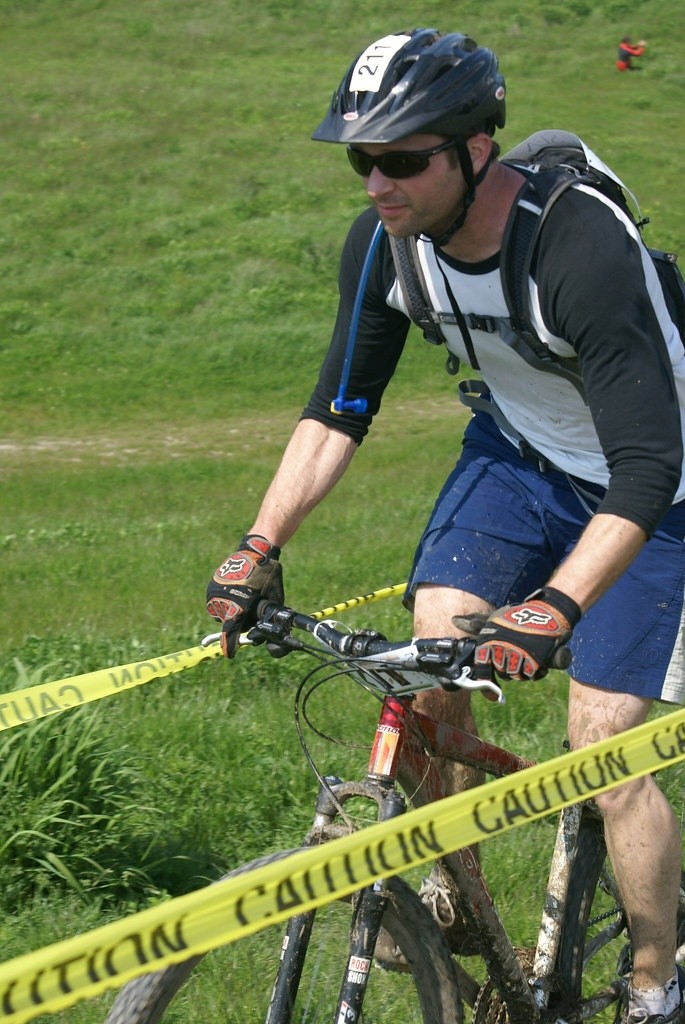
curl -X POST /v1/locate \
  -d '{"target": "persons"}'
[206,29,685,1023]
[617,34,647,71]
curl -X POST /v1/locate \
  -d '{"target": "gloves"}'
[452,586,583,701]
[205,534,285,659]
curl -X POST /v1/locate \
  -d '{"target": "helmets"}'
[311,28,506,144]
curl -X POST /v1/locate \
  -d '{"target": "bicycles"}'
[105,598,685,1024]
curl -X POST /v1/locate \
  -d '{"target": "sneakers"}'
[611,962,685,1024]
[373,863,498,974]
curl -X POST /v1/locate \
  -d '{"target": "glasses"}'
[347,134,470,180]
